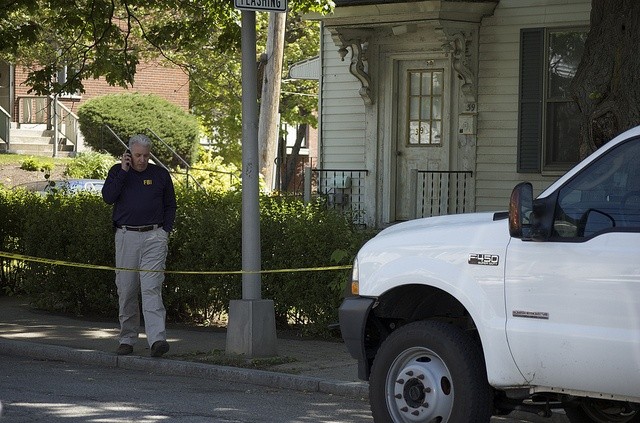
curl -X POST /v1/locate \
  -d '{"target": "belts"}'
[115,224,153,232]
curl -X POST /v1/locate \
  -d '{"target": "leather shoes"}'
[151,341,169,357]
[116,344,133,355]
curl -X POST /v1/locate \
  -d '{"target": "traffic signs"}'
[233,0,289,12]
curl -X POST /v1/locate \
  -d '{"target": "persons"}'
[100,135,176,355]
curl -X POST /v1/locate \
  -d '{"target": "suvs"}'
[335,123,640,421]
